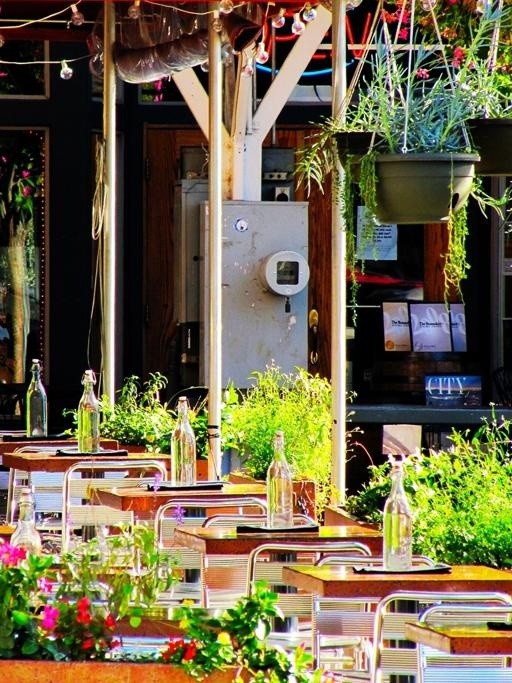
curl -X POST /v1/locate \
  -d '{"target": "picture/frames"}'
[0,127,50,391]
[0,39,51,101]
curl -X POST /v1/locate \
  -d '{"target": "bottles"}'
[77,369,99,454]
[266,429,294,530]
[9,488,41,556]
[170,395,197,489]
[25,358,48,439]
[382,462,413,571]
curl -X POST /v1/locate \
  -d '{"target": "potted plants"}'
[63,357,450,529]
[284,1,512,329]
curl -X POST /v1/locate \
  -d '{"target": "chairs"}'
[0,429,512,683]
